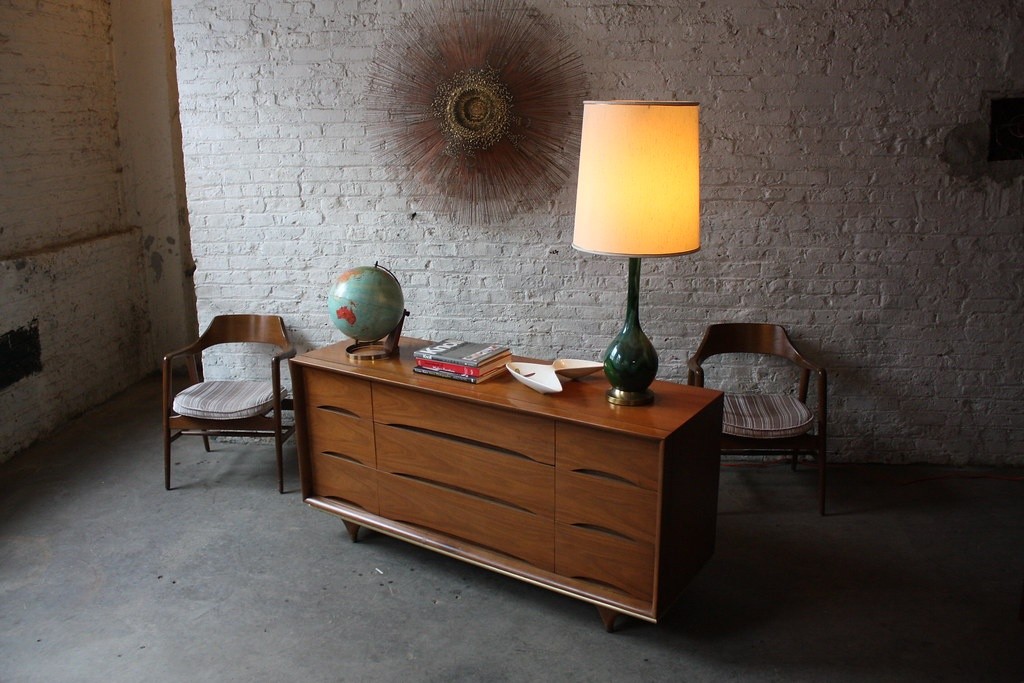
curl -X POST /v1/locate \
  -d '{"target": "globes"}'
[328,261,410,361]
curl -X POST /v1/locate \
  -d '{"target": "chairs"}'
[689,323,827,516]
[163,315,297,494]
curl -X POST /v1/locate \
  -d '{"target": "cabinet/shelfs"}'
[290,337,726,633]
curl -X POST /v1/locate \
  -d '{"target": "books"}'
[413,339,513,385]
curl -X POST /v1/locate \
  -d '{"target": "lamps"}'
[572,99,701,406]
